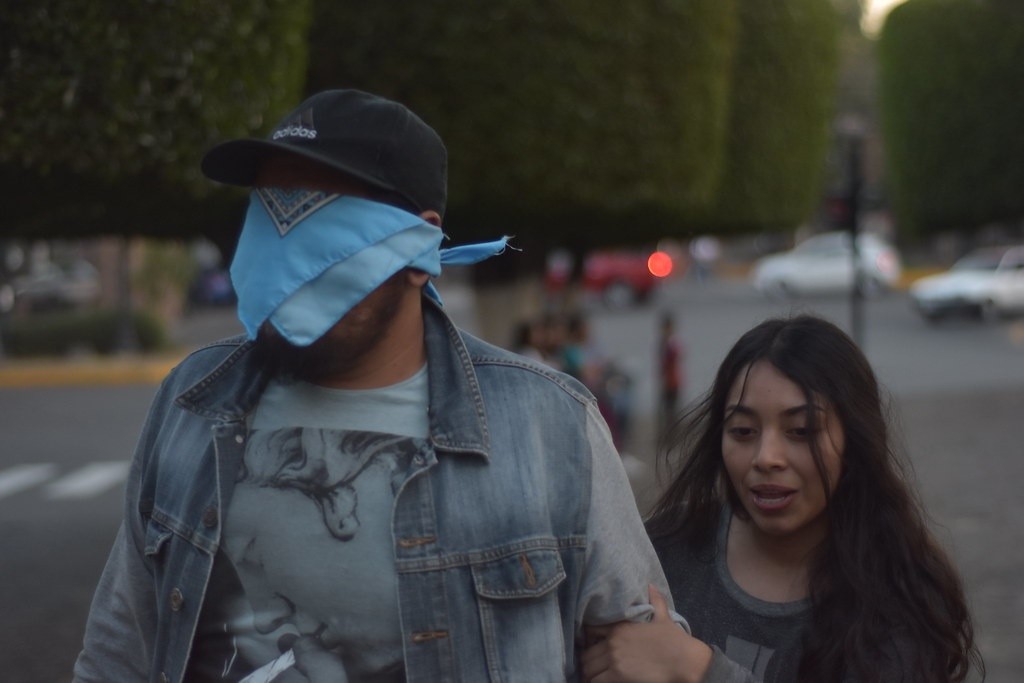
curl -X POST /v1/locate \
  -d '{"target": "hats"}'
[200,89,449,222]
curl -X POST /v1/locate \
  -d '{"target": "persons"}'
[506,308,685,452]
[65,86,690,683]
[579,313,987,682]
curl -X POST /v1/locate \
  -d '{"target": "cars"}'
[910,245,1024,323]
[754,230,901,301]
